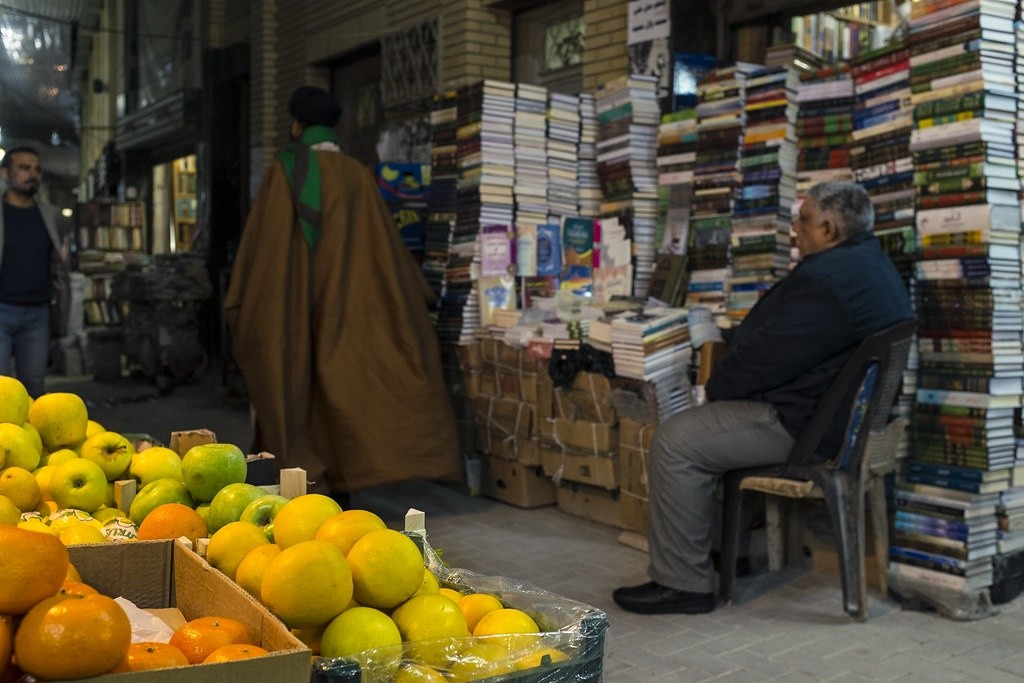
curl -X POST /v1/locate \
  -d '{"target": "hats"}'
[288,86,344,130]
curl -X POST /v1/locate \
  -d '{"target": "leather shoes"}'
[709,549,751,577]
[613,579,717,614]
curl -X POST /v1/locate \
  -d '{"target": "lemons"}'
[204,493,571,683]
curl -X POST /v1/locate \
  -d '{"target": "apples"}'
[0,376,287,544]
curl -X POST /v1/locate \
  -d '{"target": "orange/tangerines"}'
[0,466,272,683]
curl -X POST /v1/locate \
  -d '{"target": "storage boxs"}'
[14,539,607,683]
[468,338,656,534]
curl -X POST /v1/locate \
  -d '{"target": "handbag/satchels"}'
[52,272,70,340]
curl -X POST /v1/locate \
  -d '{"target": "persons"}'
[0,143,69,402]
[609,180,915,615]
[220,85,464,522]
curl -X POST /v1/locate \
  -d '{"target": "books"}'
[336,0,1024,621]
[58,155,200,383]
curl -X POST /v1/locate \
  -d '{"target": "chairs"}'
[717,316,920,621]
